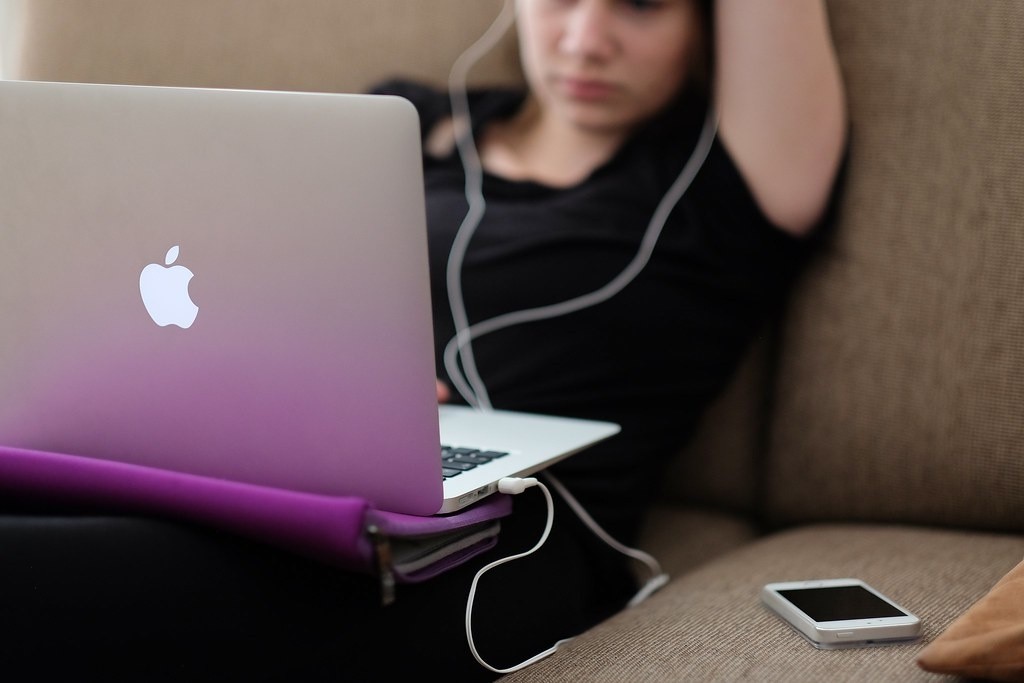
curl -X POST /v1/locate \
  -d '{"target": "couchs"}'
[23,0,1024,683]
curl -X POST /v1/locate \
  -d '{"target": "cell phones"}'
[761,578,921,643]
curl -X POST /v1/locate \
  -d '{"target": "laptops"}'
[0,80,621,517]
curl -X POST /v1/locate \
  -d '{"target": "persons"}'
[0,1,856,683]
